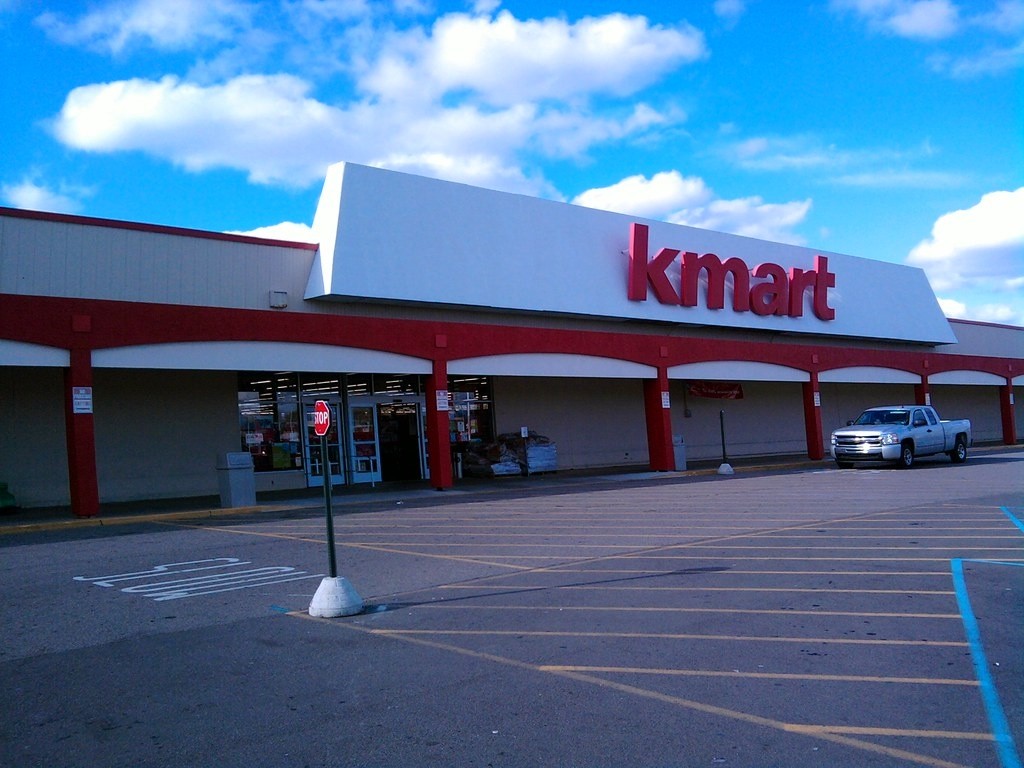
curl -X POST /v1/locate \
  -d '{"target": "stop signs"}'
[313,401,330,436]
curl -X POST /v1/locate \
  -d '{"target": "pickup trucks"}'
[830,406,972,469]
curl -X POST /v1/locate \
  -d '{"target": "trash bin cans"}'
[452,452,462,479]
[213,451,258,509]
